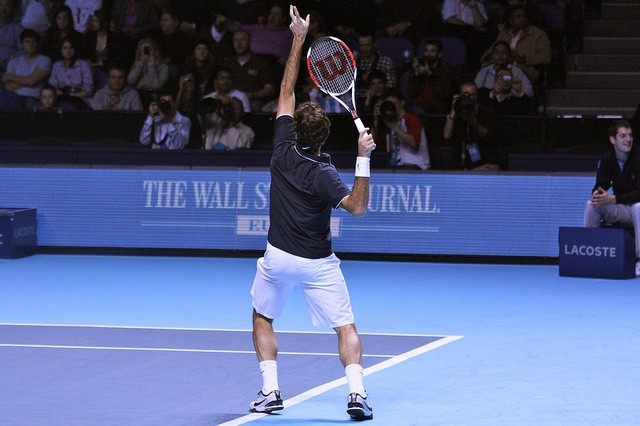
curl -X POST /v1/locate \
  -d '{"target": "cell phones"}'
[144,46,150,55]
[112,91,119,96]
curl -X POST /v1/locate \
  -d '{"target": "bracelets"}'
[354,155,371,178]
[447,114,455,122]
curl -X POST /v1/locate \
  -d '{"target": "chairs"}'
[85,68,110,91]
[358,36,416,90]
[418,36,469,81]
[57,99,80,112]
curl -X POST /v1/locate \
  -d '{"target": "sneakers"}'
[346,390,373,419]
[249,390,284,413]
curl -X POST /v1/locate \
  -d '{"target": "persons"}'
[370,88,430,171]
[247,4,378,421]
[442,78,505,173]
[197,97,255,151]
[311,1,571,111]
[3,0,288,113]
[140,92,191,151]
[583,120,640,277]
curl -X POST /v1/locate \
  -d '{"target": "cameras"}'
[416,54,436,68]
[497,74,512,83]
[222,99,233,113]
[153,99,171,112]
[454,92,476,111]
[376,100,398,123]
